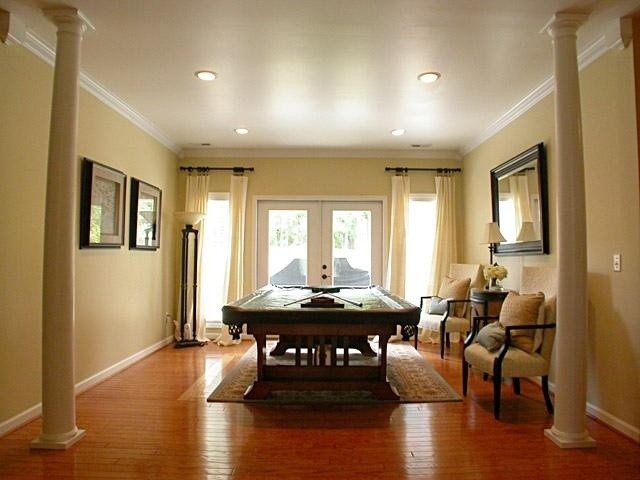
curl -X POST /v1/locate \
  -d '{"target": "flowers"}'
[483,265,508,281]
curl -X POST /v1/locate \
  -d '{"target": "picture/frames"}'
[129,177,162,251]
[79,157,127,250]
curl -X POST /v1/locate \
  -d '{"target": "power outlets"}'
[613,255,621,272]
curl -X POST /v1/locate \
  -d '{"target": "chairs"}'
[462,294,556,419]
[415,264,488,359]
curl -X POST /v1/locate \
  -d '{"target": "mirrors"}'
[490,142,549,256]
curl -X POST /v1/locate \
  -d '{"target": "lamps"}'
[516,222,537,241]
[479,222,507,265]
[172,212,207,348]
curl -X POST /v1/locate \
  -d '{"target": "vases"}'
[489,278,497,290]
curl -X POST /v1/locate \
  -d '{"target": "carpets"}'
[207,339,462,403]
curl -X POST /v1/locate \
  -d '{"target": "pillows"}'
[427,276,471,316]
[477,289,545,354]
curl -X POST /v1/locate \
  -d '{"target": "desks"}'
[221,284,422,400]
[467,288,509,380]
